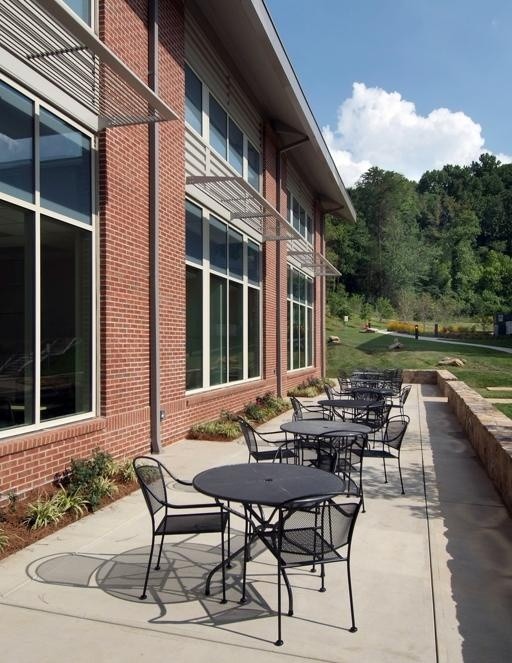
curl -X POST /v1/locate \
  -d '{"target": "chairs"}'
[337,368,404,400]
[239,492,363,646]
[353,400,393,449]
[290,395,335,422]
[272,438,339,473]
[237,416,300,464]
[385,385,412,425]
[133,456,232,604]
[313,430,369,513]
[348,414,410,494]
[324,383,353,419]
